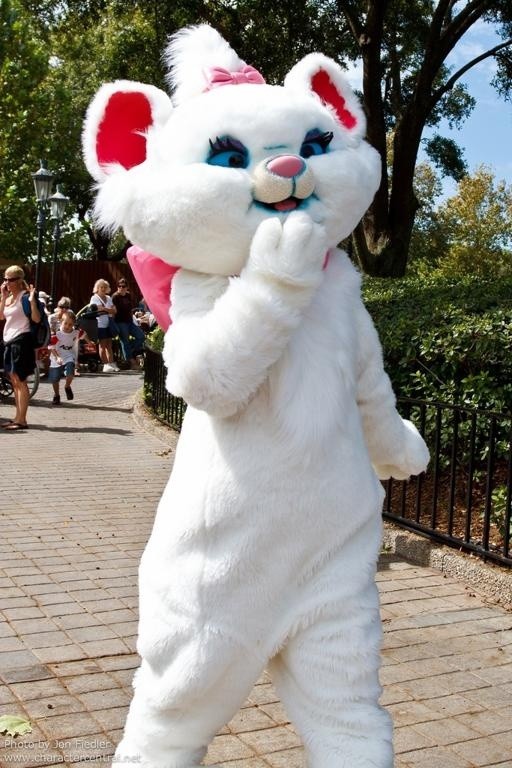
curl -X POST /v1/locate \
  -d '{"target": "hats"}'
[38,291,50,298]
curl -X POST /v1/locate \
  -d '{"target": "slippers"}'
[0,420,28,430]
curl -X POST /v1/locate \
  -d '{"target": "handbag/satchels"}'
[109,319,120,337]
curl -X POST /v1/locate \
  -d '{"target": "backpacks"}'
[22,291,51,349]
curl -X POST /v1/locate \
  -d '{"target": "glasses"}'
[59,305,69,310]
[118,284,126,288]
[2,277,20,283]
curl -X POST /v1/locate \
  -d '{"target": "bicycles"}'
[0,347,40,402]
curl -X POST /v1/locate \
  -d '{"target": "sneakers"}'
[102,362,120,372]
[52,394,60,405]
[64,385,73,400]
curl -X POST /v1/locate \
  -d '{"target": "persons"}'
[0,264,41,430]
[37,278,158,372]
[48,312,80,405]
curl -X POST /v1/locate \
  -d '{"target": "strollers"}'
[77,336,100,371]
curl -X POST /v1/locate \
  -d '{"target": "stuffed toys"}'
[80,21,429,768]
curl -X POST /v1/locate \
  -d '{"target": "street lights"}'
[45,182,71,305]
[31,157,54,290]
